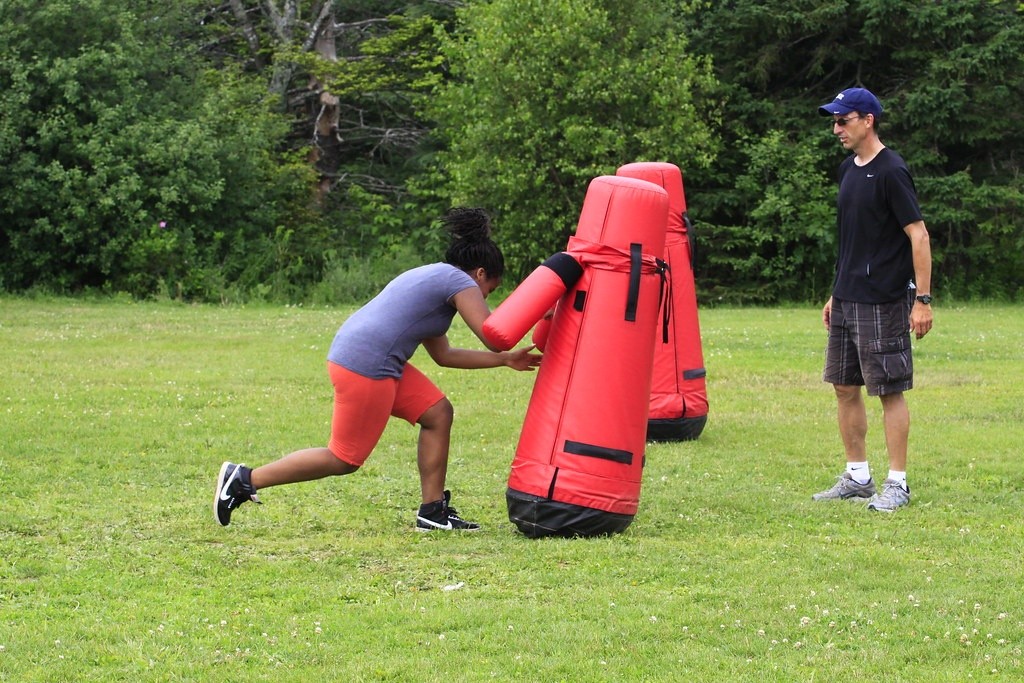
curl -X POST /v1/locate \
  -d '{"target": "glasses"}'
[831,116,864,126]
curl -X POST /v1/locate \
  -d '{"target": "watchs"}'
[916,295,933,304]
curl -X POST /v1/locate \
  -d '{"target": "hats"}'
[819,87,882,116]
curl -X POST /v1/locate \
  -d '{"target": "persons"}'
[816,88,933,514]
[216,201,541,536]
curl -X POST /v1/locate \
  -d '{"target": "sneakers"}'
[213,461,260,528]
[812,472,910,512]
[415,490,481,533]
